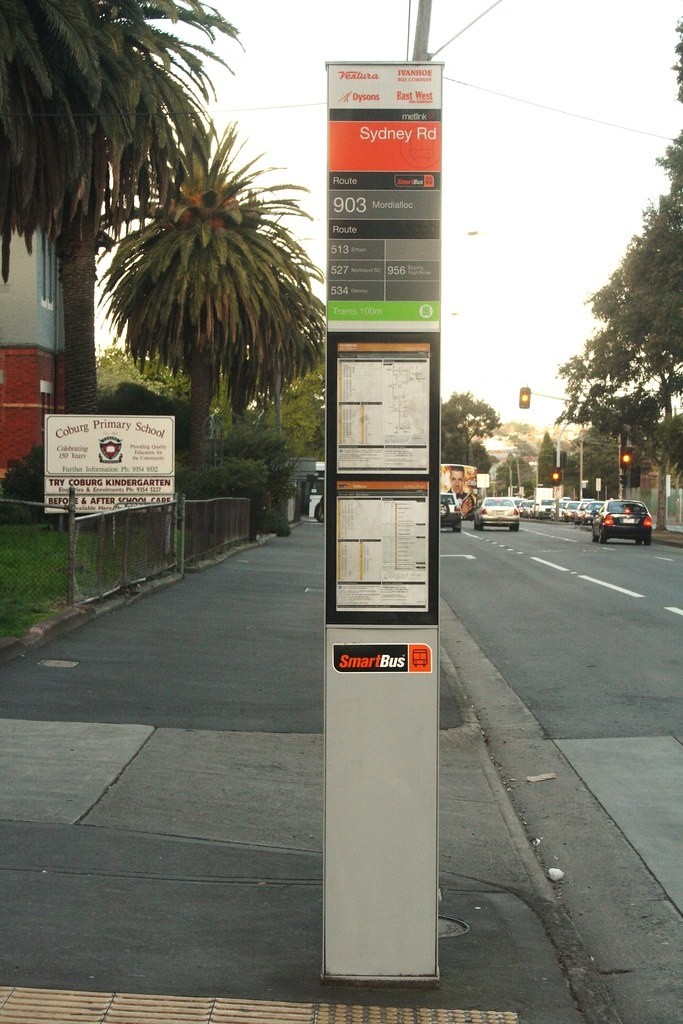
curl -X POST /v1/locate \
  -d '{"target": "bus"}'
[309,462,480,523]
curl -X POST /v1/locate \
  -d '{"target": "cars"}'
[472,498,521,534]
[590,500,653,546]
[511,496,605,526]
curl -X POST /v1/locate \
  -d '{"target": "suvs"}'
[440,493,462,533]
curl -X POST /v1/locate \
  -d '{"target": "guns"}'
[462,491,472,503]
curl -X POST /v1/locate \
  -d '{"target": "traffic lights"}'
[519,387,530,409]
[631,465,641,487]
[553,472,560,485]
[621,447,632,467]
[620,474,626,489]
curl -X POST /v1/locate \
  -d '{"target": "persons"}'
[445,465,476,520]
